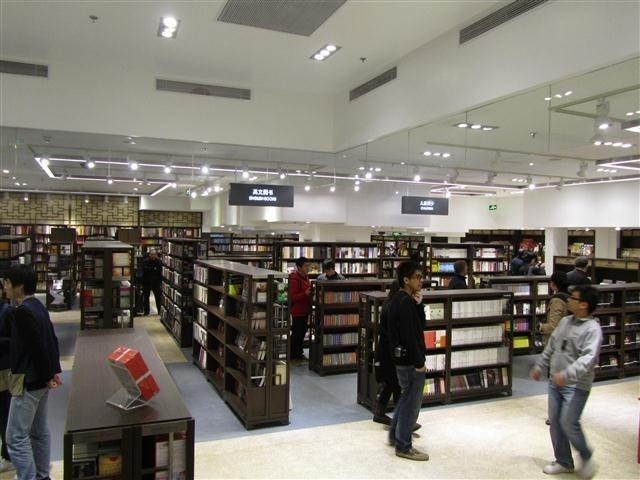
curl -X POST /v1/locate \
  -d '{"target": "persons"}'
[139,247,163,316]
[529,284,604,480]
[510,248,545,276]
[0,280,15,473]
[372,279,422,434]
[3,263,63,480]
[287,256,315,367]
[566,255,594,286]
[534,270,569,379]
[384,260,430,461]
[316,257,349,281]
[388,242,409,257]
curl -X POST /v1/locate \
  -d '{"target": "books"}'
[360,298,512,400]
[193,263,288,402]
[140,227,198,260]
[282,246,379,340]
[72,432,189,480]
[372,240,418,278]
[209,234,298,255]
[160,241,208,343]
[109,346,160,401]
[424,246,508,288]
[493,281,550,349]
[0,225,135,327]
[594,291,640,368]
[323,290,360,366]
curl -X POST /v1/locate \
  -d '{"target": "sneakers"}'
[413,423,421,432]
[543,463,575,475]
[395,447,429,460]
[372,415,392,425]
[1,459,16,473]
